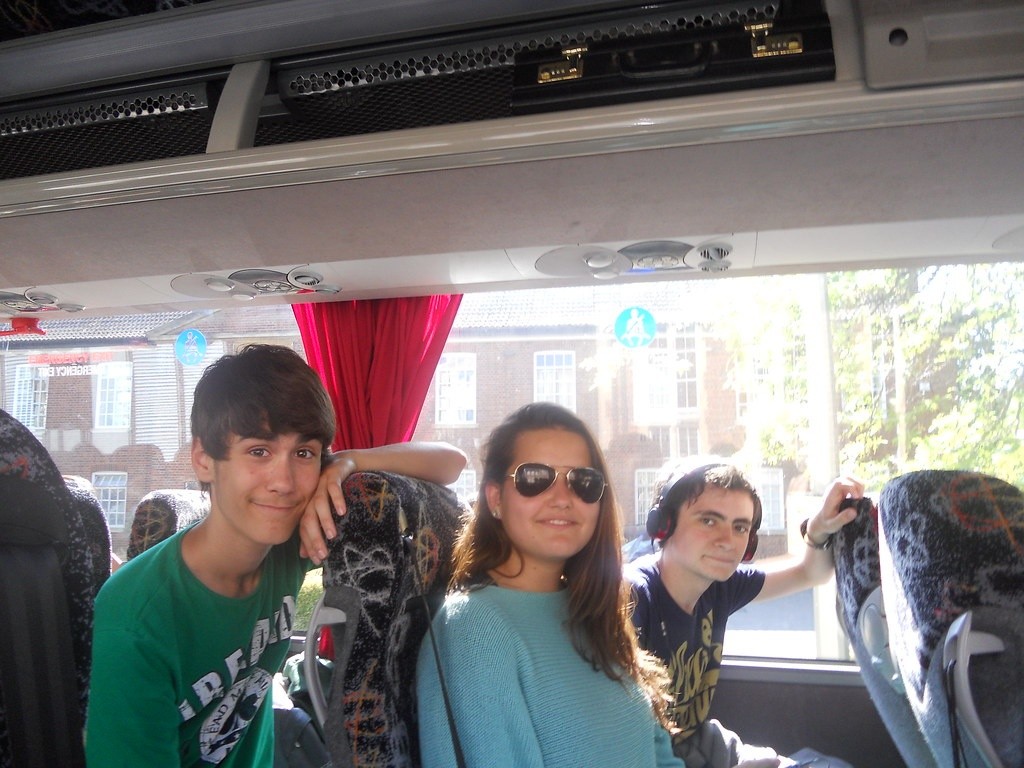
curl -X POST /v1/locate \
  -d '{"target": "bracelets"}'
[800,517,833,550]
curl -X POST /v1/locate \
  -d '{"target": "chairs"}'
[125,489,211,565]
[0,405,96,768]
[303,470,475,768]
[877,469,1024,768]
[829,496,938,768]
[59,476,113,600]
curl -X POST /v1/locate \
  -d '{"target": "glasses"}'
[504,461,610,504]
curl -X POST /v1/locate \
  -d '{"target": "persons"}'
[84,341,468,768]
[62,474,122,573]
[414,401,683,768]
[621,457,865,768]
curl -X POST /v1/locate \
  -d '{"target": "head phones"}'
[645,462,764,565]
